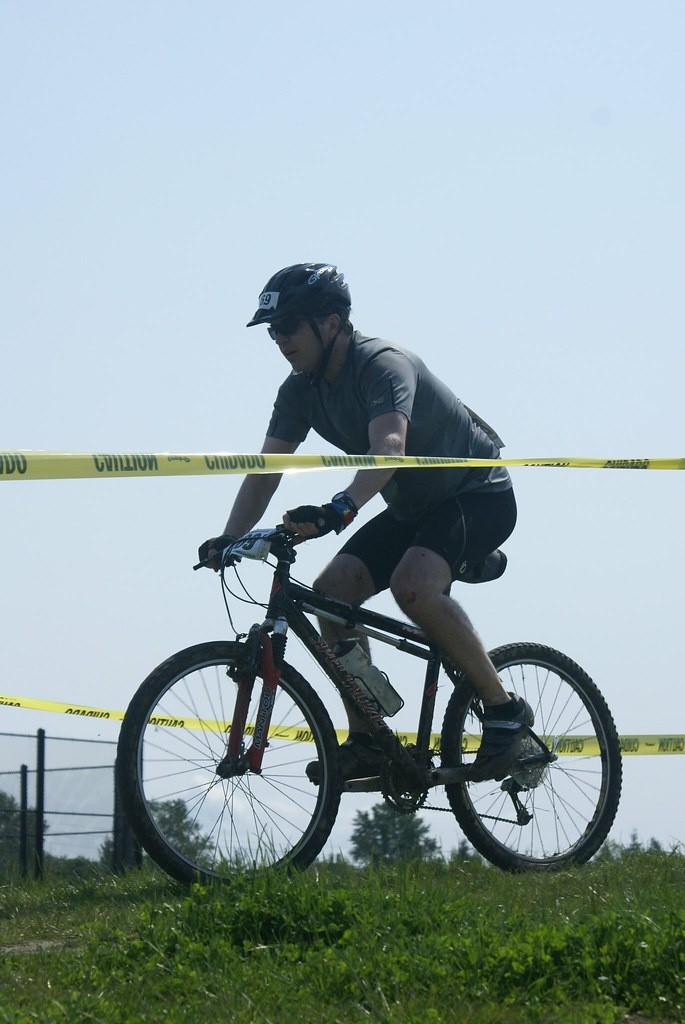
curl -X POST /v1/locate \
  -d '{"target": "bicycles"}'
[117,518,623,883]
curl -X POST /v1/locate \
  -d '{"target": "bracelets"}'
[329,500,355,535]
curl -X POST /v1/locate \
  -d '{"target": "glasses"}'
[267,313,313,340]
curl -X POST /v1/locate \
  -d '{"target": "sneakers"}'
[306,731,405,784]
[469,692,534,781]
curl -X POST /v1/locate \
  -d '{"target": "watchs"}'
[332,492,359,514]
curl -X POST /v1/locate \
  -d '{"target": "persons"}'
[200,263,534,783]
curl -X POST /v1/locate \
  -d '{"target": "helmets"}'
[247,263,350,327]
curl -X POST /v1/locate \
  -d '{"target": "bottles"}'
[332,637,401,716]
